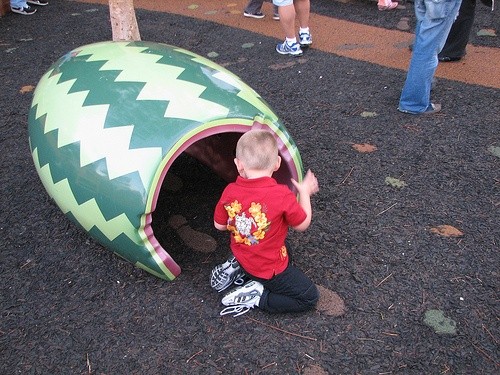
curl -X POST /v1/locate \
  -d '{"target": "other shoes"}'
[399,102,443,116]
[378,1,398,11]
[434,54,465,63]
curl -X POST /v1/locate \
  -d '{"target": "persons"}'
[409,0,478,60]
[272,0,312,57]
[210,131,320,318]
[243,0,280,20]
[377,0,398,12]
[398,0,463,115]
[10,0,49,15]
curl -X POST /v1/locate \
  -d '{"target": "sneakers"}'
[244,10,266,19]
[219,280,265,317]
[274,31,314,56]
[272,14,279,20]
[210,255,252,292]
[9,0,48,16]
